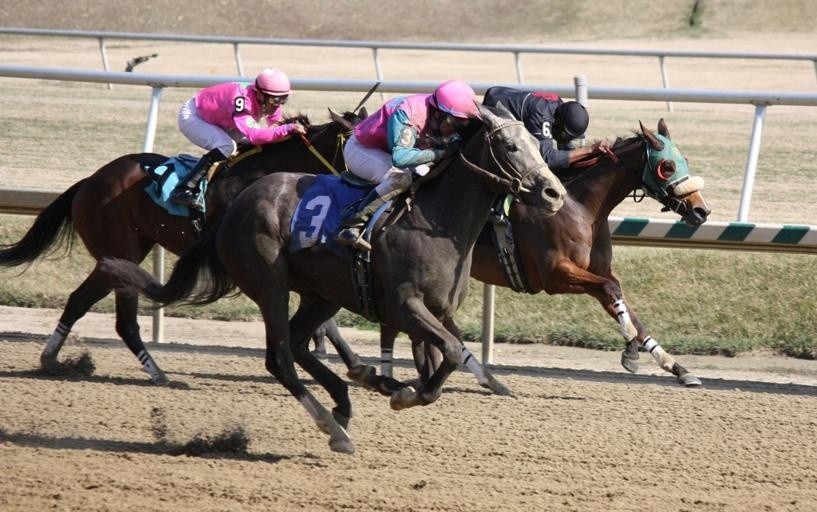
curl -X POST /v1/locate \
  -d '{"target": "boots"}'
[336,188,387,252]
[170,155,212,209]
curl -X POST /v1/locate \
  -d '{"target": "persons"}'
[171,67,307,208]
[482,86,610,225]
[333,80,481,252]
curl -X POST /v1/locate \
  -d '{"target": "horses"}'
[175,118,710,395]
[100,101,568,454]
[0,107,369,387]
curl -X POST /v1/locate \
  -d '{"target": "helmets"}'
[555,102,589,139]
[255,69,290,96]
[430,81,478,119]
